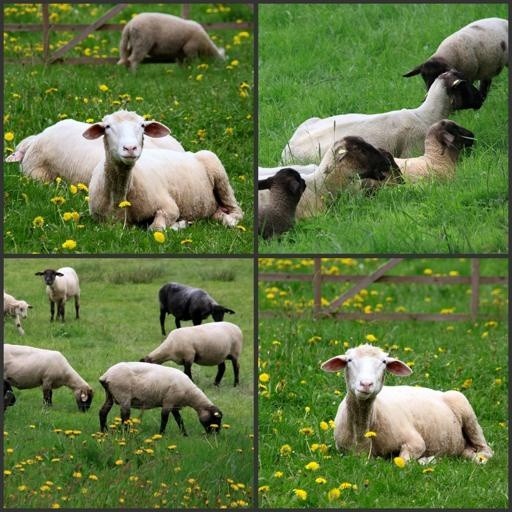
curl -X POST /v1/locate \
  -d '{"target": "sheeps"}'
[158,281,236,338]
[4,108,244,231]
[34,265,81,323]
[319,341,494,467]
[3,343,95,414]
[401,16,510,102]
[258,67,485,239]
[138,319,244,389]
[97,360,224,438]
[114,11,227,73]
[3,292,34,336]
